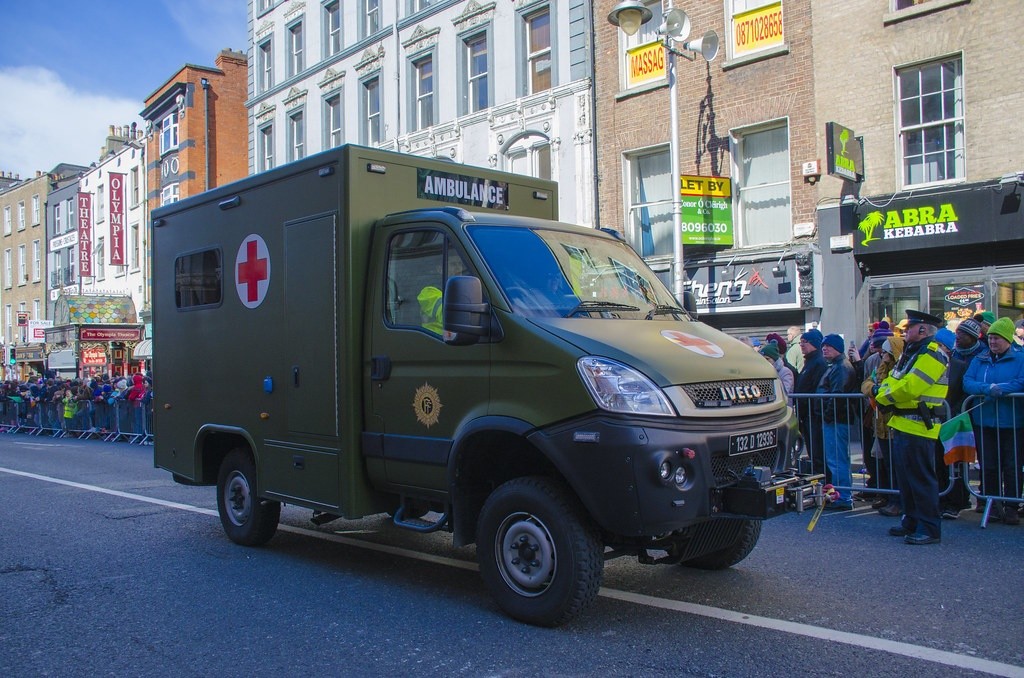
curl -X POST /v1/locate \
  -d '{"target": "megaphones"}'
[683,30,719,61]
[654,9,691,42]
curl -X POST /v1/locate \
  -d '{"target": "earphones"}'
[919,327,925,333]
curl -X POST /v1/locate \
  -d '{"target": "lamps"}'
[858,193,898,207]
[772,249,788,277]
[721,256,736,281]
[842,195,859,205]
[999,172,1024,185]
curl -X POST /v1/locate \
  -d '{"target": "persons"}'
[416,251,463,335]
[739,315,909,516]
[874,306,949,544]
[0,370,154,446]
[937,312,1024,524]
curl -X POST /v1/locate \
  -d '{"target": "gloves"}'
[872,385,880,396]
[876,400,894,414]
[989,381,1002,395]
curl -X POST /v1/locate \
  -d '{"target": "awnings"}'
[133,339,152,359]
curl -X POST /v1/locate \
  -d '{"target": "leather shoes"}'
[904,530,942,545]
[889,525,916,536]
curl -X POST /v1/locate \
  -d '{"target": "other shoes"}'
[824,503,852,510]
[1004,507,1020,524]
[976,499,986,512]
[987,505,1001,520]
[943,509,959,518]
[854,490,905,515]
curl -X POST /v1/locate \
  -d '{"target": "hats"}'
[765,333,786,354]
[935,327,957,352]
[801,328,823,350]
[820,334,844,354]
[980,311,996,326]
[760,340,779,361]
[871,320,894,348]
[903,308,943,328]
[986,317,1016,343]
[882,340,894,357]
[955,314,984,338]
[750,337,761,347]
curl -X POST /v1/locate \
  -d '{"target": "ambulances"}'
[150,143,806,629]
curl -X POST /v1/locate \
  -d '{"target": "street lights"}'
[607,0,720,311]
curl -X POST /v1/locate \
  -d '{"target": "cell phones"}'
[849,341,855,348]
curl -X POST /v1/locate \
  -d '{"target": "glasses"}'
[882,349,887,353]
[799,340,807,344]
